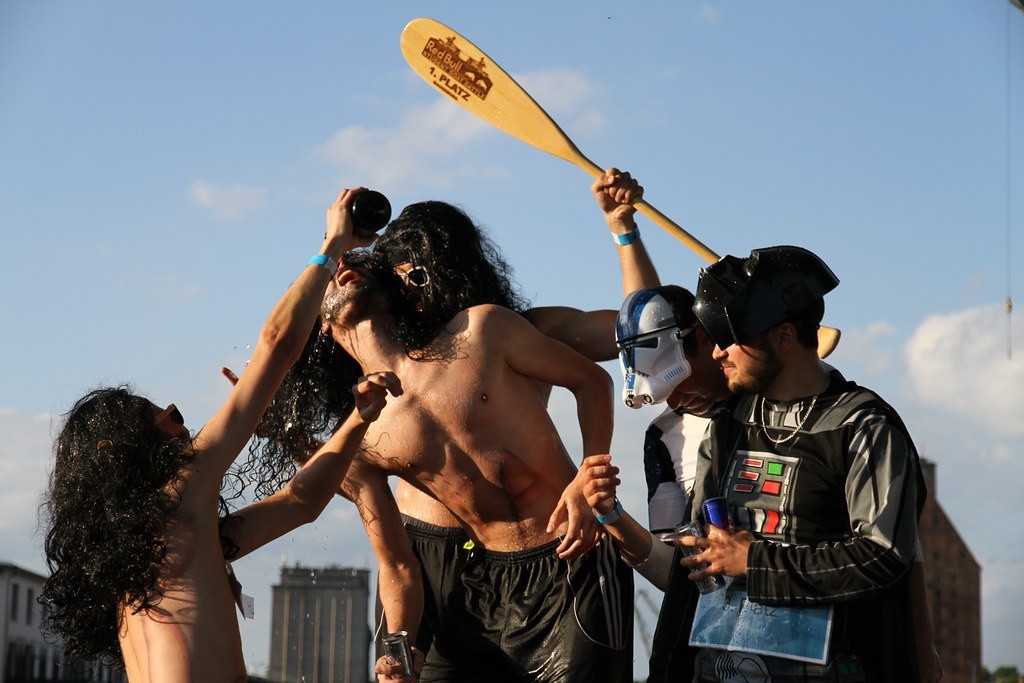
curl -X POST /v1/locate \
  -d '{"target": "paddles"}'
[399,16,844,361]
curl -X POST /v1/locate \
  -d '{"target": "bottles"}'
[324,191,391,237]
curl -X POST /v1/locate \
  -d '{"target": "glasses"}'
[347,251,366,265]
[397,266,431,288]
[153,404,184,426]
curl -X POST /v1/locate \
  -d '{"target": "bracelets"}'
[313,256,337,279]
[592,496,622,524]
[611,222,640,246]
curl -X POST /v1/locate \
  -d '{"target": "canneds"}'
[701,497,735,534]
[673,520,727,594]
[382,631,416,683]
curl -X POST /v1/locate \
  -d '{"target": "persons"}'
[39,186,943,683]
